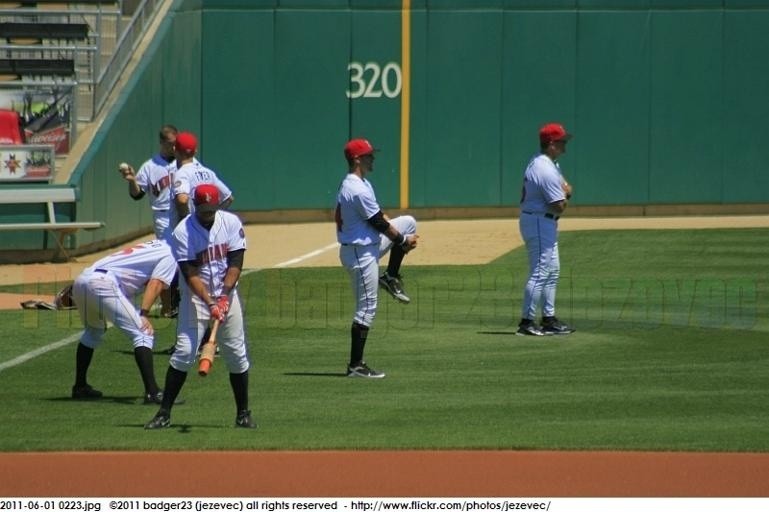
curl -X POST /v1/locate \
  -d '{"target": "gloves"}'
[209,293,229,323]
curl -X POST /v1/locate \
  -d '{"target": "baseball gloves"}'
[55,283,80,309]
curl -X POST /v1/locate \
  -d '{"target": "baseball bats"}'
[198,319,219,377]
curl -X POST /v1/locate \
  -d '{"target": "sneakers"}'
[347,361,384,378]
[516,322,552,336]
[72,384,102,398]
[161,307,178,317]
[144,391,185,404]
[540,319,576,334]
[379,272,410,304]
[236,409,257,428]
[165,341,221,358]
[144,414,171,429]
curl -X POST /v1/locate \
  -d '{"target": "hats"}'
[194,184,218,206]
[343,139,381,160]
[539,123,571,142]
[175,133,196,152]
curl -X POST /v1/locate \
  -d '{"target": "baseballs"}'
[119,162,129,171]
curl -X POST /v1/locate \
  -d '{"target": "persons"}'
[335,139,419,378]
[145,184,258,428]
[118,124,178,316]
[170,133,234,231]
[73,238,181,403]
[519,123,573,335]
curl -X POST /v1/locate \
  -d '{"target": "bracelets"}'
[221,286,231,295]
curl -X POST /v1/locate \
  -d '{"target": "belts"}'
[522,210,560,220]
[95,269,107,272]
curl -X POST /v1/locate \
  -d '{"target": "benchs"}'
[0,186,106,264]
